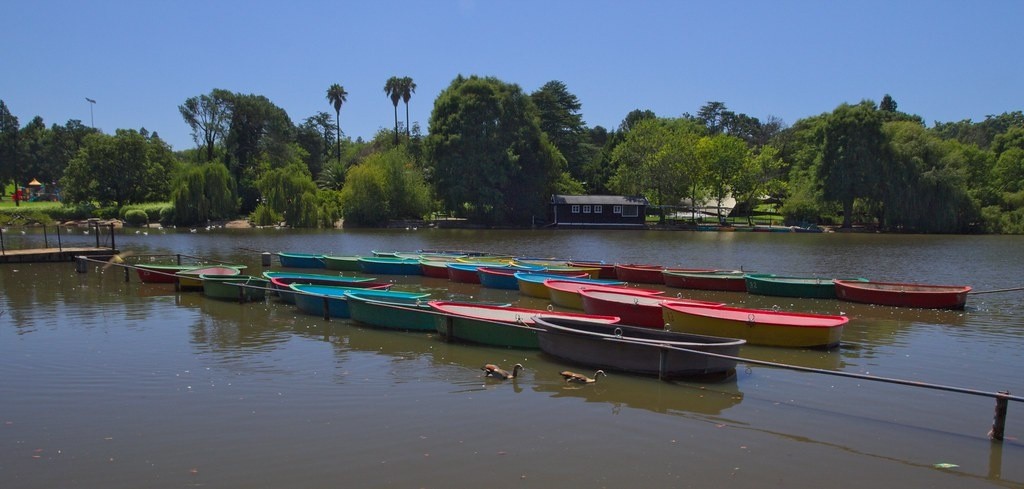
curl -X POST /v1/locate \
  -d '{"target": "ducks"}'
[559,370,607,384]
[480,362,525,379]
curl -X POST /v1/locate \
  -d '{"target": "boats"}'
[198,273,270,302]
[174,265,242,292]
[742,273,870,301]
[614,263,718,285]
[342,291,512,334]
[659,269,749,293]
[133,263,248,284]
[426,300,622,353]
[263,251,667,325]
[576,287,727,331]
[833,278,972,310]
[658,301,849,350]
[530,313,748,378]
